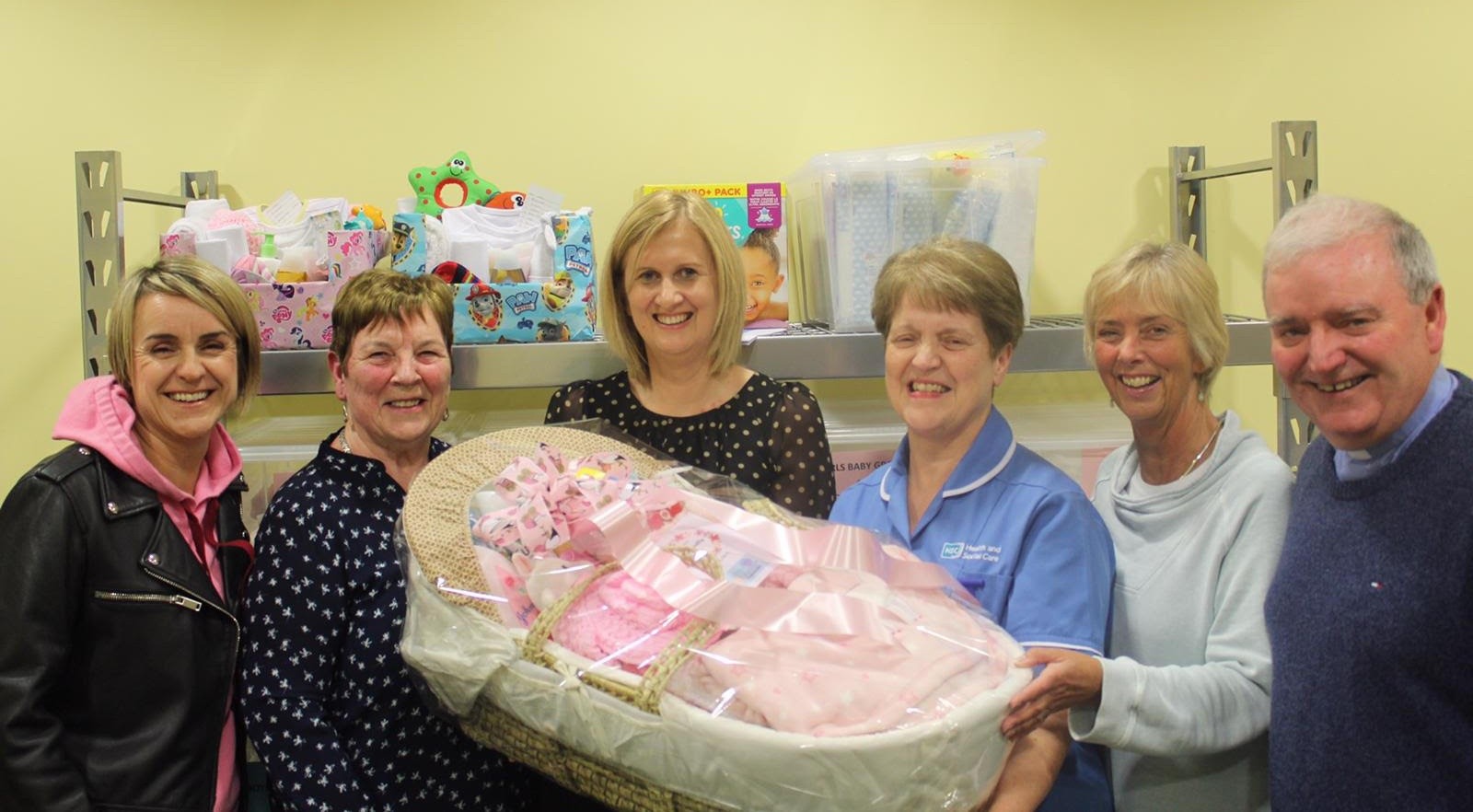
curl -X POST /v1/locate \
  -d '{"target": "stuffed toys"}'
[484,192,527,208]
[407,152,501,223]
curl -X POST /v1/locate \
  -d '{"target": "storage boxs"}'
[781,127,1055,342]
[631,179,791,329]
[992,398,1133,501]
[160,227,395,350]
[816,395,909,500]
[230,407,552,549]
[388,208,598,346]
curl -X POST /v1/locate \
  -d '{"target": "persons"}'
[546,191,837,812]
[998,239,1297,812]
[1264,191,1472,812]
[235,271,528,812]
[739,224,788,323]
[1,260,264,812]
[830,237,1119,812]
